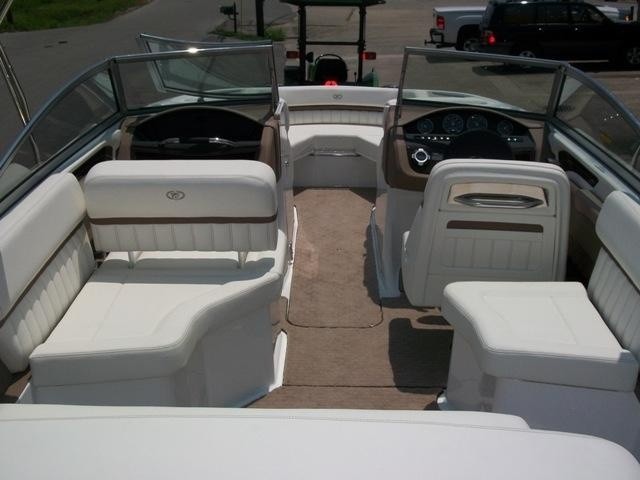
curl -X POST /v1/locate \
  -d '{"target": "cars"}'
[424,1,639,72]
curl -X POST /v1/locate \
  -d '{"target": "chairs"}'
[437,187,638,422]
[0,159,291,409]
[399,158,574,312]
